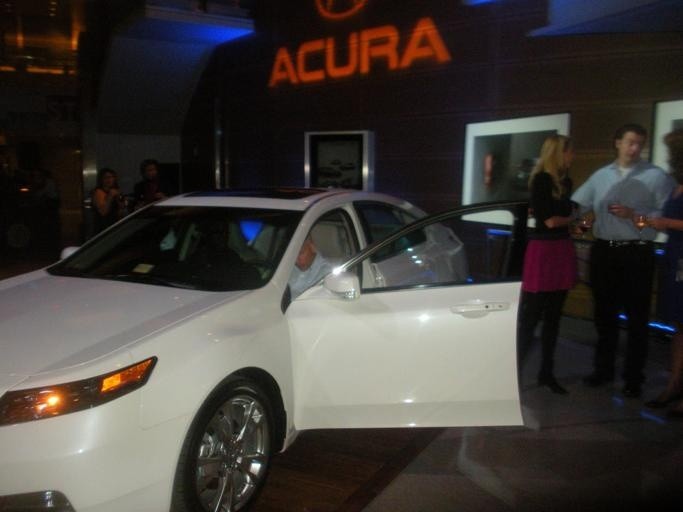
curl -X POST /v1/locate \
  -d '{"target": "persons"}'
[576,125,671,398]
[645,126,683,417]
[281,233,330,300]
[513,134,595,394]
[92,167,127,230]
[133,159,172,210]
[484,137,508,198]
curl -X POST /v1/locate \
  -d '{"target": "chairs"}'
[228,222,393,282]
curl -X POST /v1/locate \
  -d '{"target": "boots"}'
[536,360,566,394]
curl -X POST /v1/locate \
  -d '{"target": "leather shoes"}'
[623,380,641,397]
[648,393,683,407]
[584,366,613,386]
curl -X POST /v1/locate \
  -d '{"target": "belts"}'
[611,239,645,246]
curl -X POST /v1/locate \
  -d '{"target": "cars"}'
[0,186,529,511]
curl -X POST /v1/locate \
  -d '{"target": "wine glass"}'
[579,215,592,244]
[633,213,647,245]
